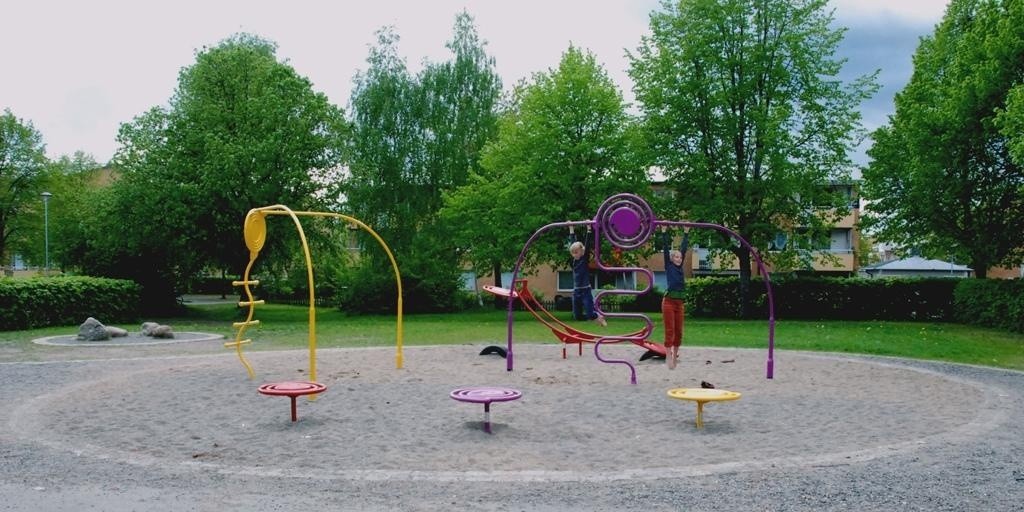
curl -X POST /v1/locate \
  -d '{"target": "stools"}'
[667,386,741,431]
[448,385,522,434]
[255,380,328,421]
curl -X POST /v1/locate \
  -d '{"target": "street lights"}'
[39,191,53,271]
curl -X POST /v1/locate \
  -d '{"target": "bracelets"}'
[586,228,591,231]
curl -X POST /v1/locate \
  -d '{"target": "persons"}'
[565,220,607,326]
[660,220,690,370]
